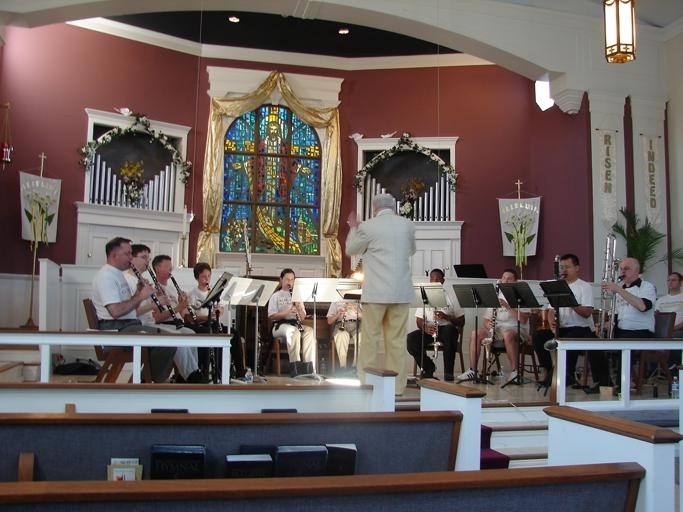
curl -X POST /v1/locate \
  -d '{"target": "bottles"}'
[671,376,678,398]
[245,368,253,383]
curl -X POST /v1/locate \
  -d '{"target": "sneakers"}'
[508,371,521,385]
[457,368,480,381]
[583,380,613,394]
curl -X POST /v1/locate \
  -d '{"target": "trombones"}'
[599,233,624,337]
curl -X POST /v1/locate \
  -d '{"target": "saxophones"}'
[544,276,566,369]
[432,307,443,360]
[481,283,503,362]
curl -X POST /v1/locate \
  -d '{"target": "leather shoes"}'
[423,366,435,378]
[187,369,201,382]
[443,373,453,381]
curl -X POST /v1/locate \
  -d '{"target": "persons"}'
[580,258,654,397]
[326,281,362,374]
[456,268,529,387]
[407,268,465,382]
[656,271,682,378]
[90,236,177,383]
[533,254,597,389]
[345,193,416,398]
[123,243,246,385]
[268,269,317,377]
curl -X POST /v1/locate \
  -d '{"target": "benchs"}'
[0,393,682,512]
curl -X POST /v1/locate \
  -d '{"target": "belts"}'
[336,319,356,324]
[98,319,136,323]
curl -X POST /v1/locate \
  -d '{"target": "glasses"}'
[134,254,151,261]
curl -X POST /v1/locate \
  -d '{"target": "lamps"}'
[601,0,639,65]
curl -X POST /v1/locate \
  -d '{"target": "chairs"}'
[483,307,680,397]
[413,325,465,376]
[81,297,247,382]
[262,318,356,376]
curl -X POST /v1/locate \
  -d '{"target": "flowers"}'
[499,208,541,270]
[17,186,60,256]
[69,109,194,211]
[347,129,462,220]
